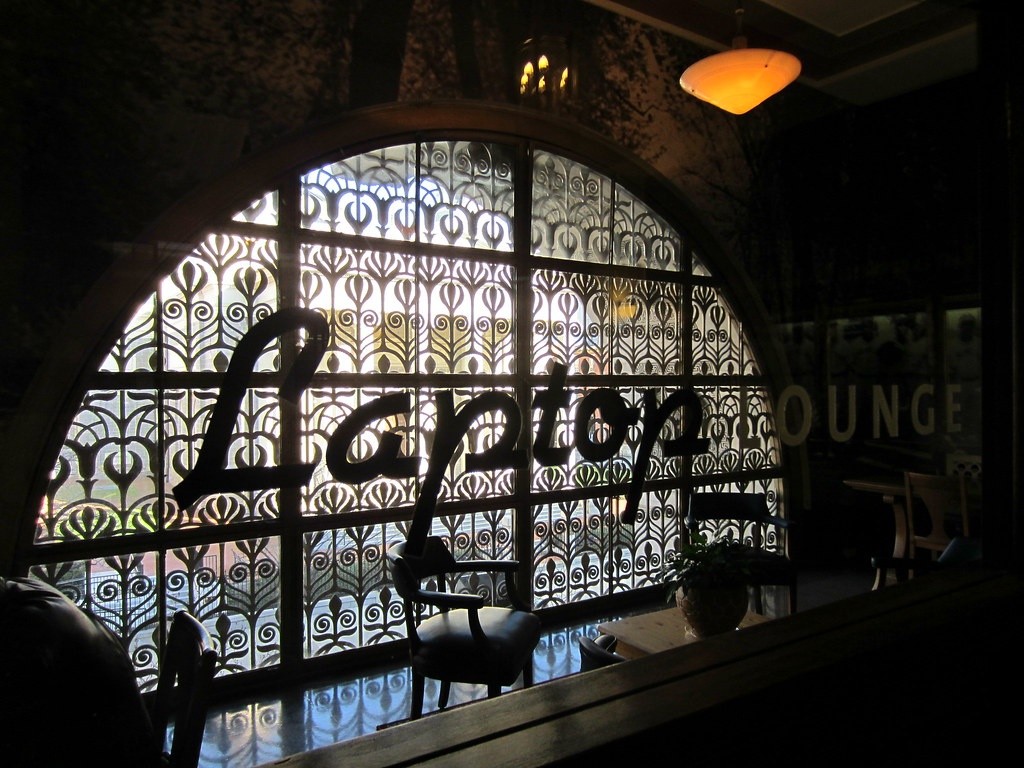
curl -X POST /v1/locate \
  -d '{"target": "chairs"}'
[150,611,217,768]
[947,453,983,496]
[904,470,970,579]
[387,536,543,721]
[578,631,626,672]
[685,492,799,615]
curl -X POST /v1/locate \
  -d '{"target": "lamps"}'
[678,9,802,115]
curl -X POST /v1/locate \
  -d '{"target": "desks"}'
[594,606,775,661]
[843,467,984,591]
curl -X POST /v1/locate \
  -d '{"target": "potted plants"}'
[641,528,769,639]
